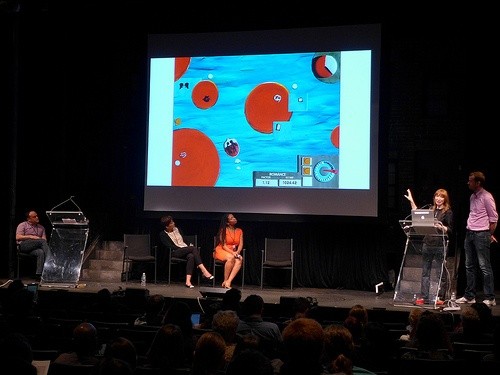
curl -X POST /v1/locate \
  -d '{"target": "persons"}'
[16,210,51,281]
[405,189,453,307]
[160,215,214,289]
[455,172,498,307]
[213,213,243,289]
[0,273,500,375]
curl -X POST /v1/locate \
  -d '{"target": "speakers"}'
[279,297,308,306]
[199,291,227,299]
[126,288,149,298]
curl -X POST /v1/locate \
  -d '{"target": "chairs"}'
[122,234,158,285]
[17,245,34,278]
[168,235,201,286]
[213,237,248,290]
[261,238,295,290]
[0,313,500,375]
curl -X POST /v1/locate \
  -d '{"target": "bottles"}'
[451,292,456,301]
[141,273,146,286]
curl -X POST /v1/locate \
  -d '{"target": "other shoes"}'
[36,275,41,281]
[185,282,195,289]
[222,283,232,289]
[416,299,429,305]
[202,275,214,279]
[435,300,444,305]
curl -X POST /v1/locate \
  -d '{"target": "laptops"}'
[410,208,439,233]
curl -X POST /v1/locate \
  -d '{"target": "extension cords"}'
[442,306,462,312]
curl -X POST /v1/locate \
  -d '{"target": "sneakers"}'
[455,296,476,304]
[483,299,496,306]
